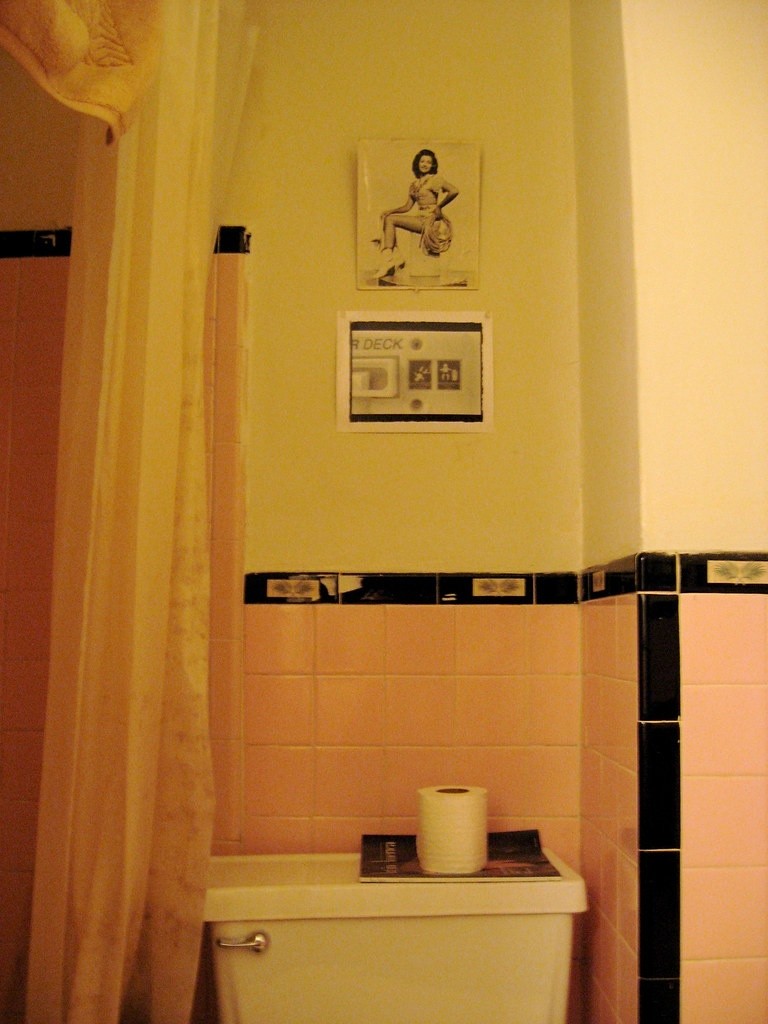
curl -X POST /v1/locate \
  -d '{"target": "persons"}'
[373,149,459,281]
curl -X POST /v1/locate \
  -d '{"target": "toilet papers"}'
[415,784,489,876]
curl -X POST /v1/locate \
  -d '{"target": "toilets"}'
[195,841,590,1024]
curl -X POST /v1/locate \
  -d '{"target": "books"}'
[357,829,563,887]
